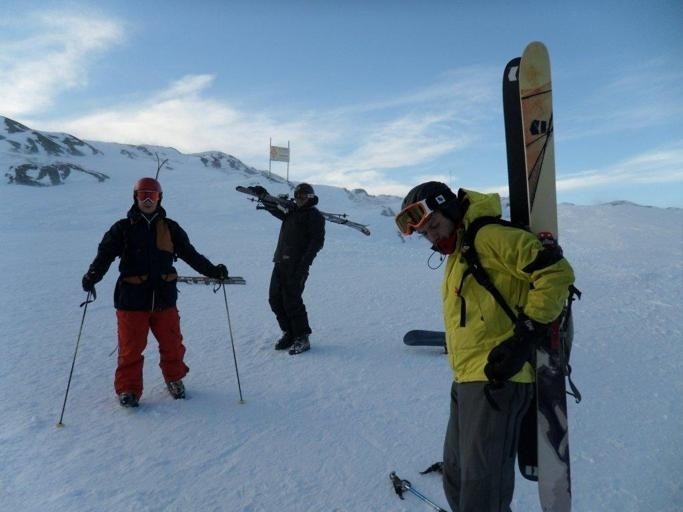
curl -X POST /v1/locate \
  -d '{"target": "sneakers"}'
[168,381,184,397]
[119,392,138,406]
[275,331,292,349]
[289,333,310,354]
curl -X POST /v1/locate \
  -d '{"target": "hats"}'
[294,184,314,199]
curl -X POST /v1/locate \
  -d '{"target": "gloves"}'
[212,263,228,280]
[248,186,268,199]
[83,265,102,290]
[484,336,527,382]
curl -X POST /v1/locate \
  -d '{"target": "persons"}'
[245,181,324,356]
[392,181,575,512]
[81,178,229,408]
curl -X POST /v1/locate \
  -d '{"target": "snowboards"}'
[404,330,448,353]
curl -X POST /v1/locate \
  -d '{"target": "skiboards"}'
[236,185,370,236]
[502,41,573,512]
[177,276,246,285]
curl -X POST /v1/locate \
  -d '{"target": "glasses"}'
[395,192,455,234]
[135,191,159,204]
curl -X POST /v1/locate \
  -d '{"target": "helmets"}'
[134,178,161,193]
[401,182,458,220]
